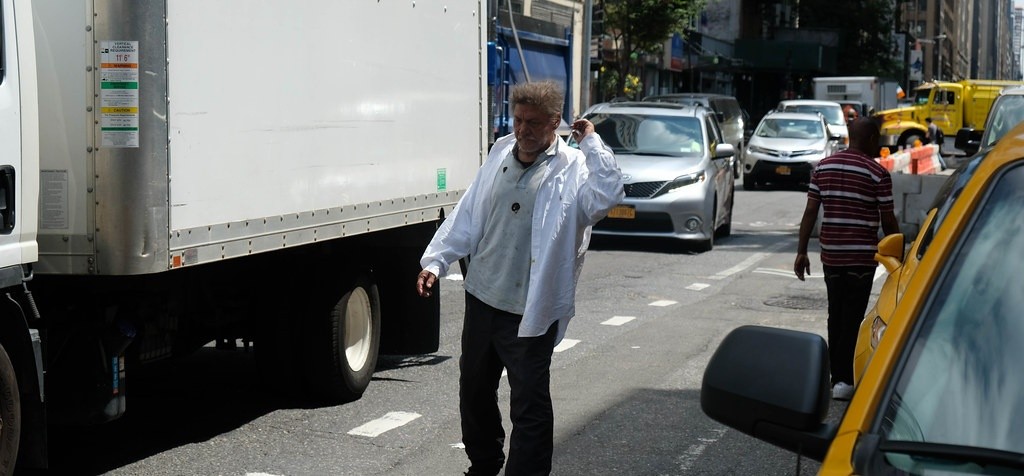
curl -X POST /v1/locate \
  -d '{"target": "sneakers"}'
[832,382,854,401]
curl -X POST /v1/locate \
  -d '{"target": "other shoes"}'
[461,462,505,476]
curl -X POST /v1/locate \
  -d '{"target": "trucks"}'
[813,76,880,127]
[484,0,593,157]
[0,0,489,475]
[872,78,1024,151]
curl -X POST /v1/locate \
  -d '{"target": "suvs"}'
[742,110,841,191]
[953,85,1024,151]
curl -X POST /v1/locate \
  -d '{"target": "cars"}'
[701,121,1024,476]
[853,139,997,391]
[564,95,735,255]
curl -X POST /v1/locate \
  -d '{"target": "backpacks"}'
[936,127,944,144]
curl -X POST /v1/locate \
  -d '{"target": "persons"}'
[925,117,947,171]
[416,80,626,475]
[794,117,903,399]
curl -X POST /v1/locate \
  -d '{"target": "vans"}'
[777,100,849,153]
[642,94,744,180]
